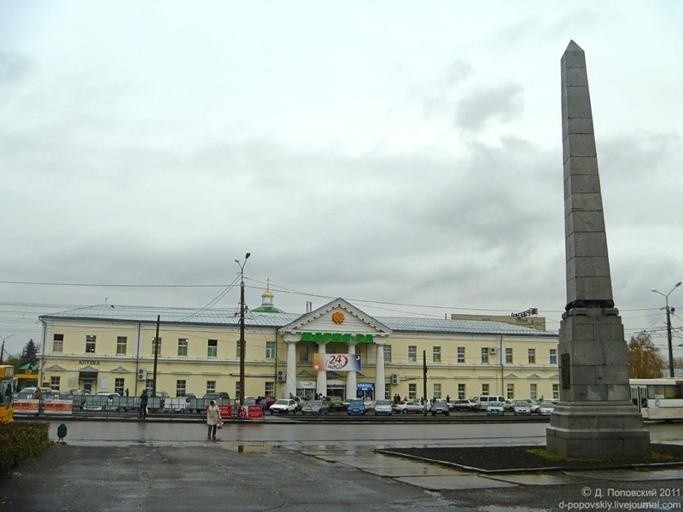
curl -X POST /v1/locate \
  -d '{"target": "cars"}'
[15,387,123,412]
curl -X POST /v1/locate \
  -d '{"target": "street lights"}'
[235,252,250,405]
[651,282,681,377]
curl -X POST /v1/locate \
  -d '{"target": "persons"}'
[445,393,451,403]
[205,399,222,441]
[136,388,148,419]
[430,395,437,417]
[4,382,12,411]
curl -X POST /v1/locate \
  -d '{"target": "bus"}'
[0,365,15,424]
[629,377,683,421]
